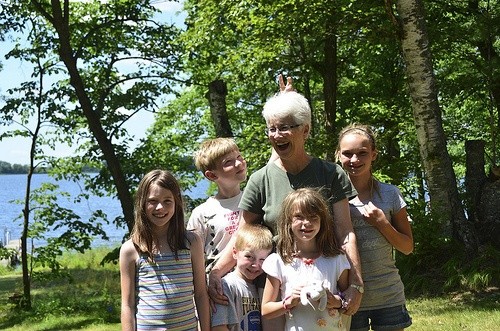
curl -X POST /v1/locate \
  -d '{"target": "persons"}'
[117,168,212,330]
[333,122,414,330]
[208,90,364,317]
[185,73,294,289]
[260,187,352,331]
[210,223,274,331]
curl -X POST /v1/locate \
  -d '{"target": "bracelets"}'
[337,292,348,310]
[283,295,291,311]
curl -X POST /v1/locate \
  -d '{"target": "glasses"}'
[268,125,300,133]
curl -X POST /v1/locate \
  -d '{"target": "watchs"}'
[348,284,364,294]
